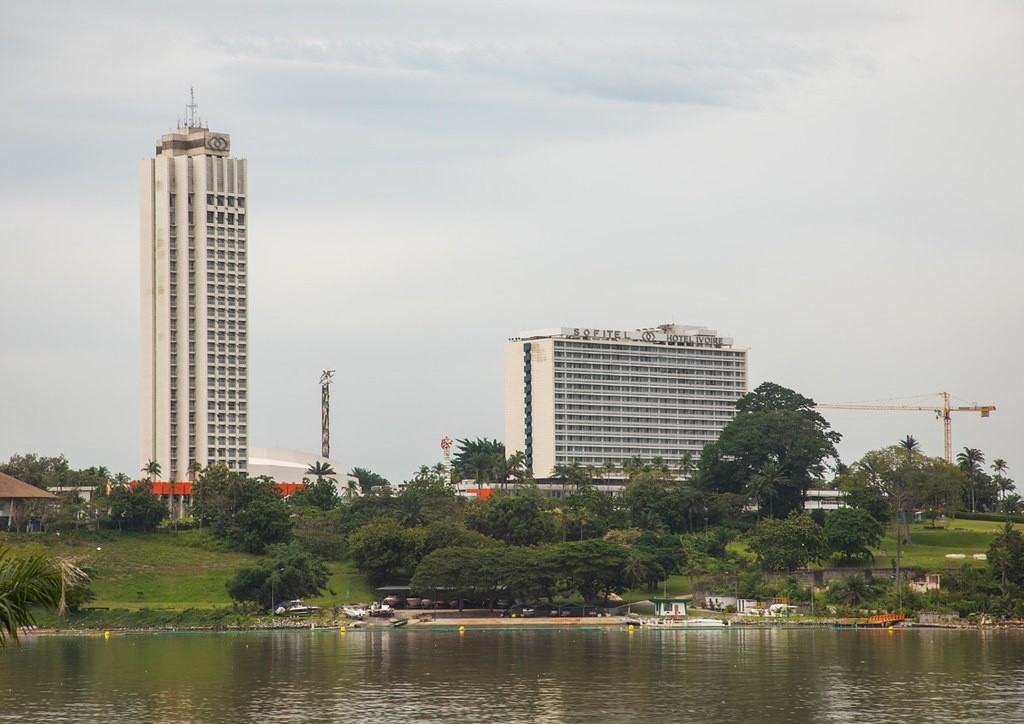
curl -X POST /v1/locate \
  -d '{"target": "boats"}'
[523,607,535,617]
[638,616,730,626]
[284,599,320,617]
[384,594,400,607]
[627,612,905,629]
[561,610,571,617]
[549,608,558,617]
[406,597,458,610]
[344,604,367,620]
[368,602,394,617]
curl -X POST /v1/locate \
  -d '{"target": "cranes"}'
[811,392,997,464]
